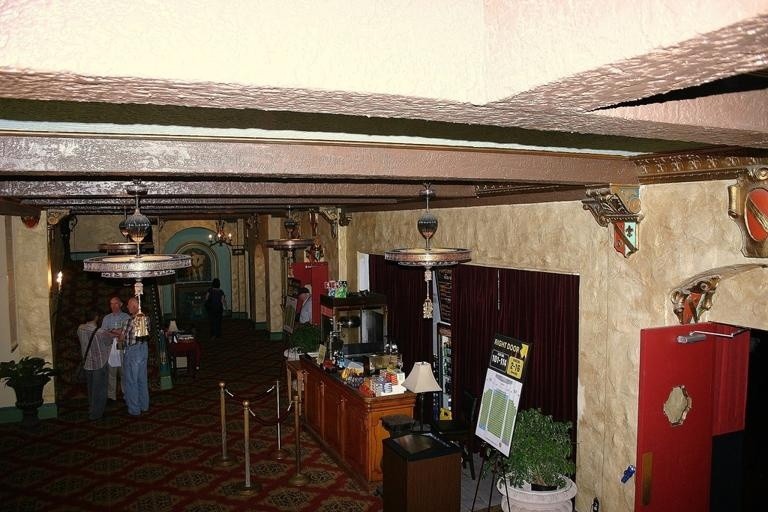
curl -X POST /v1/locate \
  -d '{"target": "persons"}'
[101,295,130,412]
[77,309,113,423]
[203,278,227,338]
[117,297,151,419]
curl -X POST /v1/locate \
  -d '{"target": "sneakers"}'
[87,412,106,425]
[106,397,149,418]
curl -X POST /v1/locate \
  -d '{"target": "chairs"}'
[431,388,478,480]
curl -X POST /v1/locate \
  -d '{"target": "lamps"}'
[384,182,472,319]
[266,207,314,278]
[84,179,195,337]
[207,214,233,247]
[96,208,154,287]
[50,271,63,298]
[401,361,443,433]
[168,320,178,344]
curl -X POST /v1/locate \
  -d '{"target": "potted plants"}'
[481,408,577,512]
[0,355,75,428]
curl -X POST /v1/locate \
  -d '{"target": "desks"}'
[165,334,200,372]
[286,361,305,416]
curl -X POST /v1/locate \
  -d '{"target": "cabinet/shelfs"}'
[302,358,417,492]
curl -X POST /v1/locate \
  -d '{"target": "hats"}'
[295,288,309,295]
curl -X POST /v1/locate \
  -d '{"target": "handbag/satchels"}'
[75,365,86,384]
